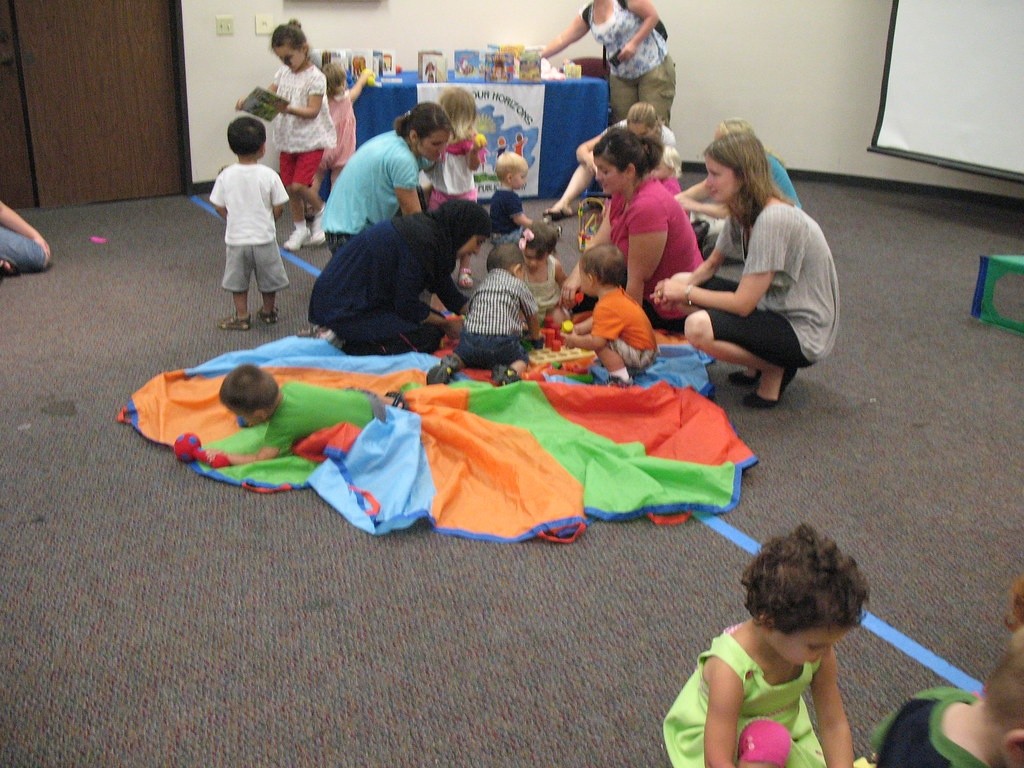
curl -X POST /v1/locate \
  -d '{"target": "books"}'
[311,44,544,84]
[239,86,290,122]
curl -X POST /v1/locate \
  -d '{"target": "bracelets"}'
[685,285,694,307]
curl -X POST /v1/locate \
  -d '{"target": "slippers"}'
[543,207,572,221]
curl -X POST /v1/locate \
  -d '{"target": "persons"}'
[420,87,489,291]
[661,523,869,768]
[318,100,455,256]
[868,576,1024,768]
[209,116,289,329]
[206,363,408,465]
[539,0,675,126]
[648,134,840,409]
[0,200,51,274]
[425,102,710,389]
[674,118,800,266]
[298,199,490,357]
[235,20,325,252]
[303,63,374,219]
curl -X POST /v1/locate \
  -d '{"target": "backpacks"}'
[582,0,668,43]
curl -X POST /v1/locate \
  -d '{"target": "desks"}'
[316,70,609,202]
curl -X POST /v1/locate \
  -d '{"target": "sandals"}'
[216,311,251,332]
[257,305,279,325]
[385,392,408,411]
[457,268,474,288]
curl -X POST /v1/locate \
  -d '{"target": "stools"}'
[971,254,1024,337]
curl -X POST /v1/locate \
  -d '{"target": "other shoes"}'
[605,375,636,389]
[426,354,460,387]
[308,208,326,246]
[728,368,762,388]
[743,366,797,409]
[702,233,718,259]
[284,228,311,252]
[311,324,344,352]
[490,364,521,384]
